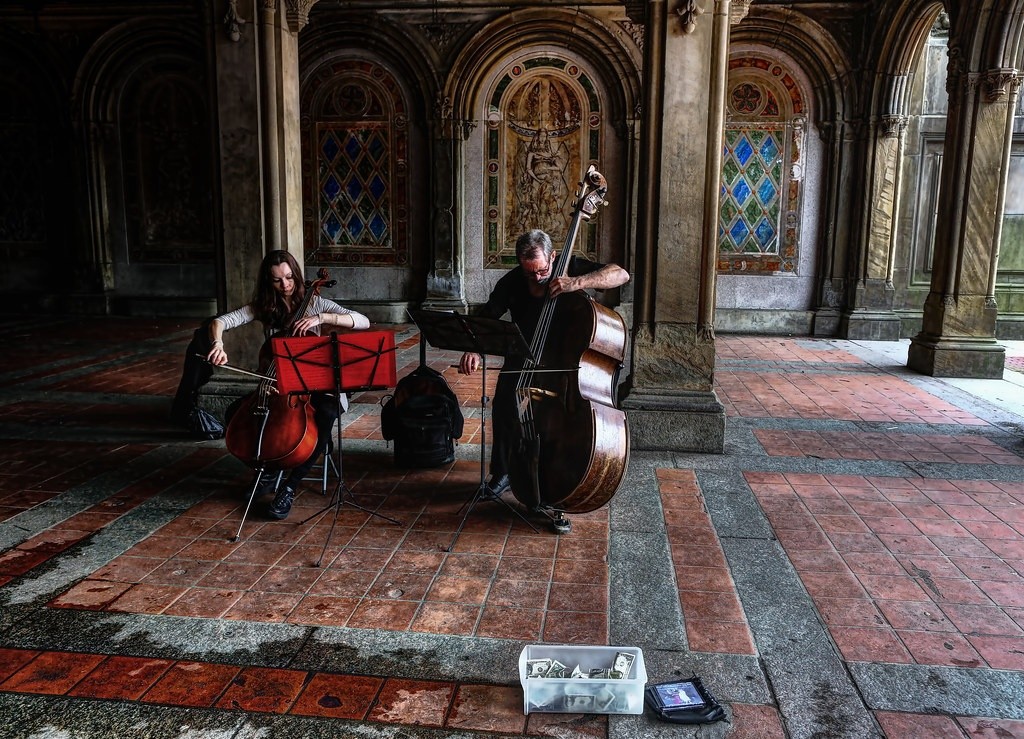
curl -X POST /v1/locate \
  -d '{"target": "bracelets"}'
[211,339,224,348]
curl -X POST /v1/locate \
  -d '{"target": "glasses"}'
[519,262,550,278]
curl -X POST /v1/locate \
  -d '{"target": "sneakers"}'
[268,481,297,520]
[247,473,277,500]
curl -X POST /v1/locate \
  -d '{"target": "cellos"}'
[492,165,630,519]
[224,268,338,542]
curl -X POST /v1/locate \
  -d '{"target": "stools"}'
[272,431,338,496]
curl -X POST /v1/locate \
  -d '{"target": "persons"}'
[206,249,372,520]
[459,228,630,534]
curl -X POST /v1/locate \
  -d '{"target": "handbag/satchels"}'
[379,364,464,469]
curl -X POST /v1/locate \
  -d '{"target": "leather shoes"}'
[483,472,511,498]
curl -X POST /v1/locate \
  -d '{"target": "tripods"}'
[406,310,540,553]
[270,330,405,567]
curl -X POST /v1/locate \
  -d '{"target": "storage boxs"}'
[518,640,649,719]
